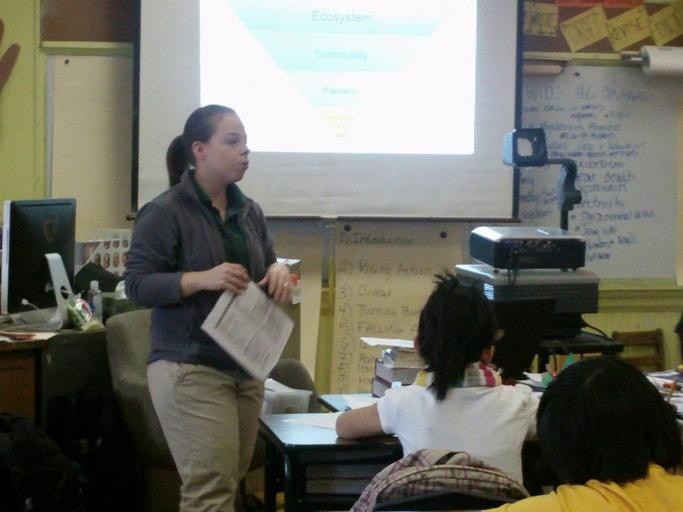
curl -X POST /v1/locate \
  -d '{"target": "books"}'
[370,347,424,398]
[200,276,294,380]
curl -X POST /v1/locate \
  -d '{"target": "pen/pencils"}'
[667,378,679,404]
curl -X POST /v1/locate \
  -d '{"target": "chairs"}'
[261,362,321,417]
[613,323,667,375]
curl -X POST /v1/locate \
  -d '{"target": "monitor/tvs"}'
[0,198,88,330]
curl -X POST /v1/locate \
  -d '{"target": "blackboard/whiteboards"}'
[330,221,463,395]
[41,42,683,292]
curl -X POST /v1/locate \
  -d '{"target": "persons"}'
[123,104,295,512]
[485,355,683,511]
[336,270,541,486]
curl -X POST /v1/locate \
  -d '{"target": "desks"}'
[252,406,404,511]
[0,256,301,511]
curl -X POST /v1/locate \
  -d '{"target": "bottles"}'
[87,280,104,326]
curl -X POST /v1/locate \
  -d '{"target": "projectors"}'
[469,226,585,268]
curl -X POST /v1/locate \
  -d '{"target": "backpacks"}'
[349,447,530,512]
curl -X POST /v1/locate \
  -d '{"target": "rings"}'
[283,281,289,287]
